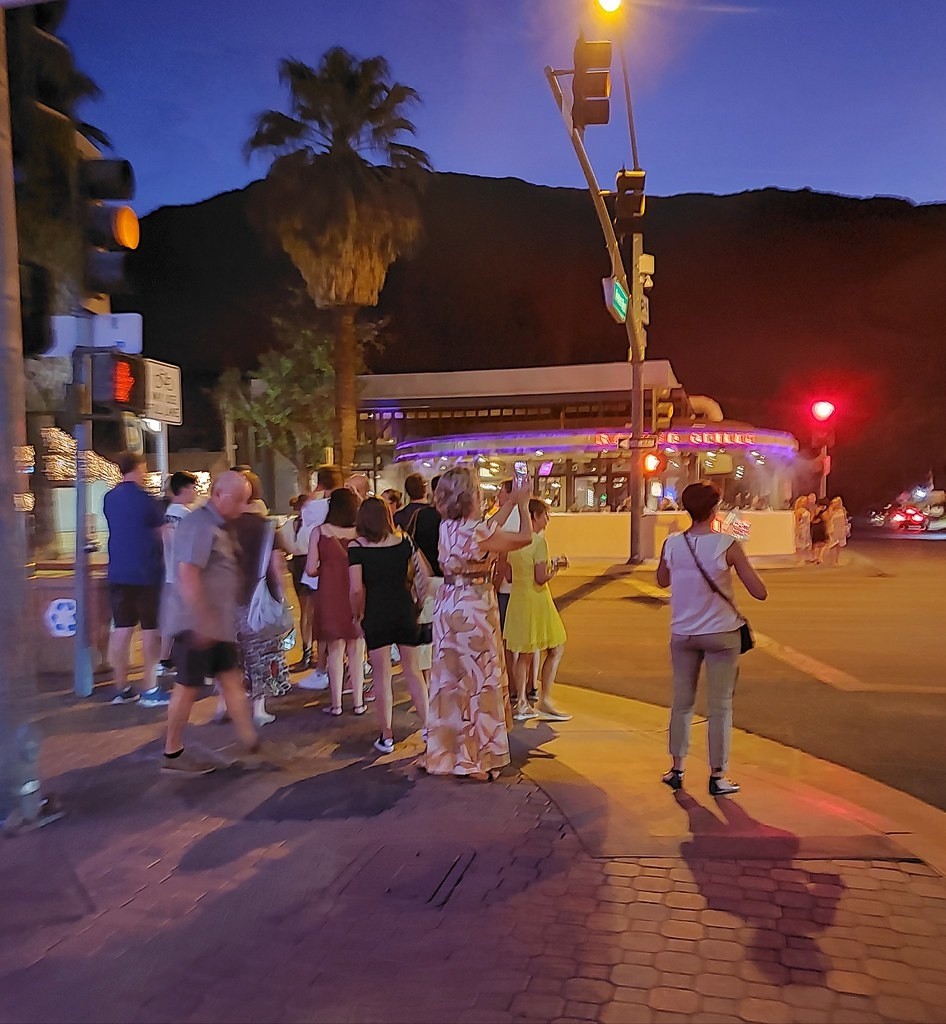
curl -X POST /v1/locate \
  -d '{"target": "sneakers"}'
[373,732,395,752]
[138,685,171,707]
[160,744,216,775]
[422,727,429,742]
[111,685,141,704]
[298,669,330,689]
[246,734,297,764]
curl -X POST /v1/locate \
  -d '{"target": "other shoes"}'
[154,658,275,726]
[509,687,539,701]
[298,646,401,701]
[533,699,572,720]
[0,792,69,835]
[512,699,540,720]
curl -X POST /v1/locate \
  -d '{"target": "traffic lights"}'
[573,35,612,131]
[4,0,78,189]
[90,351,148,409]
[64,128,141,314]
[650,384,675,434]
[615,161,646,237]
[644,452,661,475]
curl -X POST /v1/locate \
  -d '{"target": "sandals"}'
[321,705,342,716]
[353,704,368,716]
[709,775,741,795]
[661,766,684,789]
[454,770,501,782]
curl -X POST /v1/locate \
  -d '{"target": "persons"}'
[783,493,852,567]
[617,491,774,514]
[104,450,570,782]
[656,481,768,793]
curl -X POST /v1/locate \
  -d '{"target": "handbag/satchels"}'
[416,575,447,624]
[739,623,753,653]
[247,520,293,641]
[410,547,434,606]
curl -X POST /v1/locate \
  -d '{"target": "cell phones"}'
[514,458,530,487]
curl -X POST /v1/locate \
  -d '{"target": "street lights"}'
[813,399,834,500]
